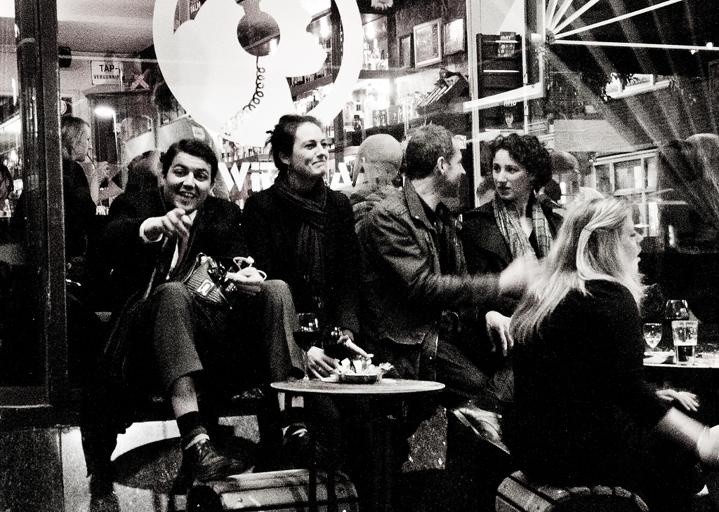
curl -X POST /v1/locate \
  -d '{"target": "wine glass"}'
[642,322,664,365]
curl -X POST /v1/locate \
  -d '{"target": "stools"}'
[493,470,649,511]
[184,463,361,511]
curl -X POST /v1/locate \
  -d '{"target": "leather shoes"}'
[176,440,234,482]
[276,422,315,470]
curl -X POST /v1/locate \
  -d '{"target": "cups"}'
[671,318,698,366]
[664,298,690,332]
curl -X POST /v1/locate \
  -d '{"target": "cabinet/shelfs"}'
[156,0,398,190]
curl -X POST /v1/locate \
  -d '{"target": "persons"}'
[355,124,558,512]
[79,137,317,494]
[0,113,718,387]
[241,115,435,512]
[496,187,719,512]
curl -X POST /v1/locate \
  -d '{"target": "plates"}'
[340,374,377,385]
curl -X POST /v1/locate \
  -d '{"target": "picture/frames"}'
[398,16,465,71]
